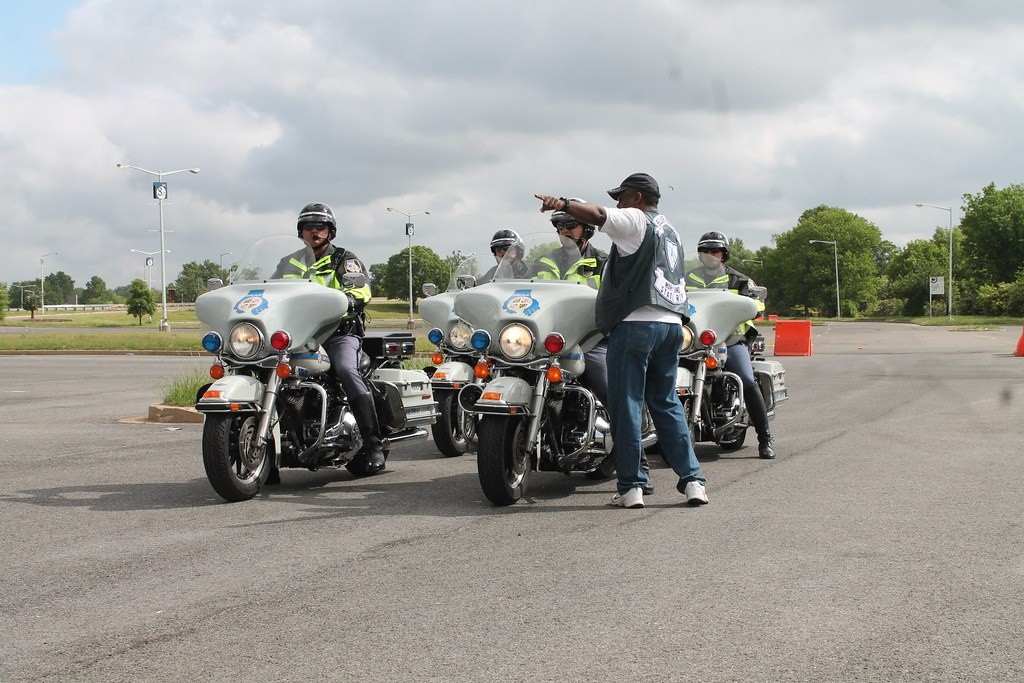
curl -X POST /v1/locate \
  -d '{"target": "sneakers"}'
[610,490,646,509]
[683,480,708,506]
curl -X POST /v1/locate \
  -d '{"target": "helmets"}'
[697,231,730,263]
[297,203,337,242]
[489,230,524,258]
[549,198,595,240]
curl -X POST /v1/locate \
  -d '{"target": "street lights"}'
[742,259,765,320]
[220,251,233,281]
[915,203,952,322]
[808,240,841,318]
[129,247,170,320]
[40,253,60,313]
[116,164,200,331]
[386,208,431,329]
[9,285,37,310]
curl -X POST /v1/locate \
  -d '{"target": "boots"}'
[640,455,653,495]
[745,384,776,459]
[349,392,385,471]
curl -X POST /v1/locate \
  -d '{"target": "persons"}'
[477,229,532,286]
[528,198,654,495]
[684,230,776,460]
[535,173,708,507]
[272,203,387,471]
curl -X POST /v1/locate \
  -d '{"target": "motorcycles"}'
[419,233,790,507]
[195,234,439,502]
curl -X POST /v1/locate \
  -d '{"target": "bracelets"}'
[560,197,569,212]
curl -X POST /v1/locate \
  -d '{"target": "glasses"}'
[555,220,581,230]
[495,246,513,254]
[700,249,723,254]
[301,225,329,231]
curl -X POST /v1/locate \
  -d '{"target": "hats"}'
[606,173,661,201]
